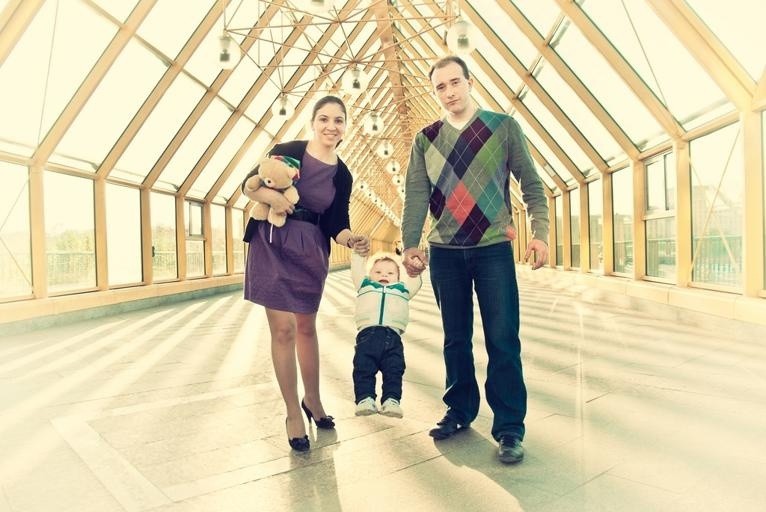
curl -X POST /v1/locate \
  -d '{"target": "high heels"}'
[301,397,335,430]
[286,417,309,451]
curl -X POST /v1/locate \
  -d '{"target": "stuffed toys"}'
[244,155,302,227]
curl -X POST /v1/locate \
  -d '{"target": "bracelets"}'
[346,237,353,250]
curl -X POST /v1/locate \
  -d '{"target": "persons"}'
[400,55,552,465]
[351,239,425,419]
[241,95,370,452]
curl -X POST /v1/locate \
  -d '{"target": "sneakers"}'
[380,397,403,418]
[356,398,378,415]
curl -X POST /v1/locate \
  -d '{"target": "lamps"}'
[446,16,479,57]
[363,110,385,135]
[271,96,294,120]
[212,28,241,72]
[341,60,369,95]
[355,140,404,200]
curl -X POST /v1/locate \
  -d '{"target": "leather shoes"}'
[429,417,461,439]
[499,436,524,462]
